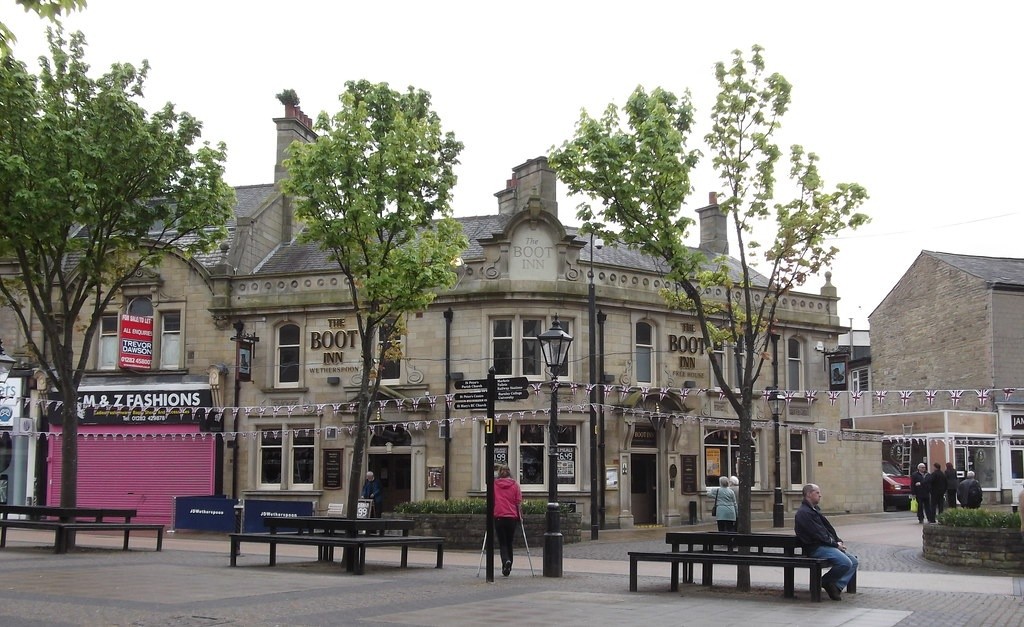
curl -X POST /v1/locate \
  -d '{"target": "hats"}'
[730,476,739,485]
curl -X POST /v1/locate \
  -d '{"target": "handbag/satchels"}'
[712,507,716,516]
[910,497,918,513]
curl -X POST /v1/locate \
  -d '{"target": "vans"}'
[882,461,913,511]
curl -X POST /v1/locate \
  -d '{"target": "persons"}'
[916,463,946,523]
[794,484,858,601]
[360,471,385,518]
[728,475,739,505]
[944,462,959,508]
[494,465,523,576]
[957,470,983,509]
[706,476,738,532]
[911,463,931,523]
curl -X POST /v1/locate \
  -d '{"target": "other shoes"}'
[826,587,842,601]
[502,559,511,577]
[821,577,840,592]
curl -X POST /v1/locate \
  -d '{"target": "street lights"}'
[767,391,787,527]
[535,313,575,578]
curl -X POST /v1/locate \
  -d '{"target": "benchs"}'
[627,531,857,603]
[0,504,165,553]
[229,515,446,575]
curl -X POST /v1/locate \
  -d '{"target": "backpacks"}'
[966,479,982,508]
